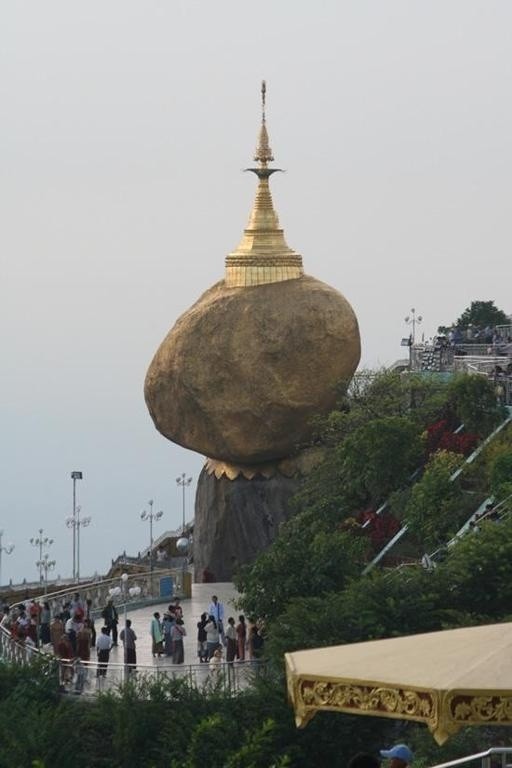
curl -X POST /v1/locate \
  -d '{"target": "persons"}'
[156,545,168,559]
[434,318,512,348]
[504,362,511,407]
[487,364,502,404]
[347,750,381,768]
[379,742,413,767]
[0,592,264,697]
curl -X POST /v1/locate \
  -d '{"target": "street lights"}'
[175,472,192,568]
[105,569,146,697]
[400,334,415,376]
[140,495,164,587]
[29,503,93,598]
[404,304,424,350]
[0,527,15,559]
[67,469,82,582]
